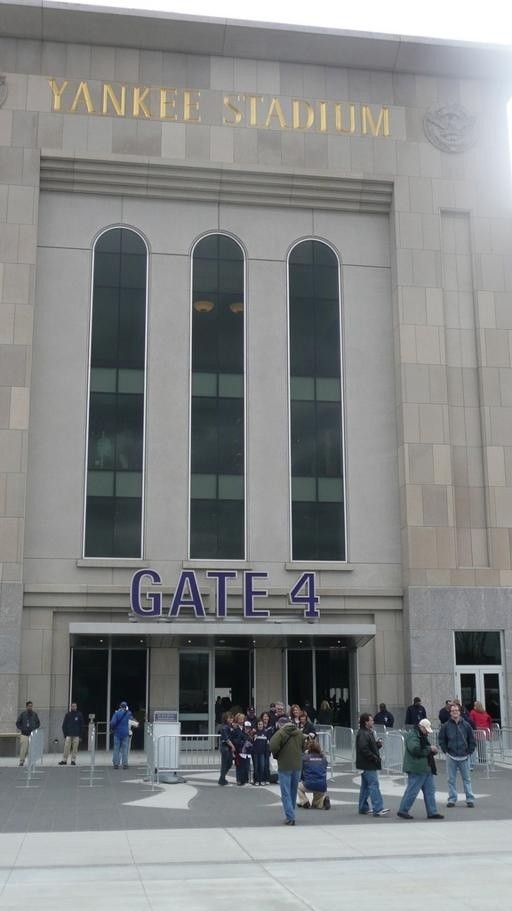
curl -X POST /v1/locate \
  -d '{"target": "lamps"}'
[193,299,216,313]
[229,302,244,313]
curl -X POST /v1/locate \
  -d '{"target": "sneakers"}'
[113,765,118,769]
[122,765,127,768]
[376,808,390,815]
[447,803,454,807]
[468,802,473,807]
[244,780,270,786]
[367,809,373,814]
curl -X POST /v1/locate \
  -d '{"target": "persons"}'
[58,702,85,766]
[118,707,139,767]
[397,697,445,819]
[298,744,331,811]
[110,701,137,770]
[16,702,41,767]
[213,695,315,786]
[318,701,335,753]
[437,699,492,808]
[355,703,394,816]
[271,717,306,824]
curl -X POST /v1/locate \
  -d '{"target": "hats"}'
[119,702,127,708]
[419,718,433,733]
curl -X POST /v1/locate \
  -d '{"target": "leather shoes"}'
[58,761,67,764]
[284,818,295,825]
[324,796,330,809]
[297,802,310,807]
[398,812,413,818]
[70,761,75,765]
[428,814,443,818]
